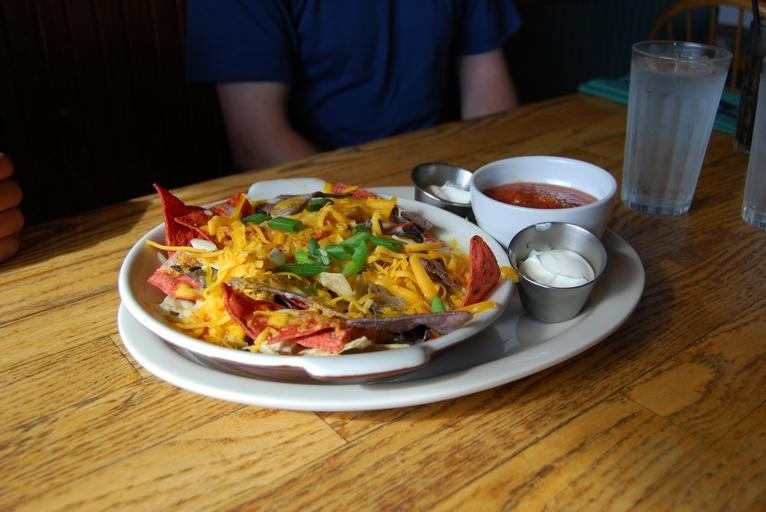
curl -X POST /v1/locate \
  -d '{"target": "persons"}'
[184,0,524,171]
[0,153,24,265]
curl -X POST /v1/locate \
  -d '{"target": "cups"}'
[621,38,766,230]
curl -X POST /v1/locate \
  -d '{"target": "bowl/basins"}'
[471,154,618,253]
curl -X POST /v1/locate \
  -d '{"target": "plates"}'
[119,177,647,412]
